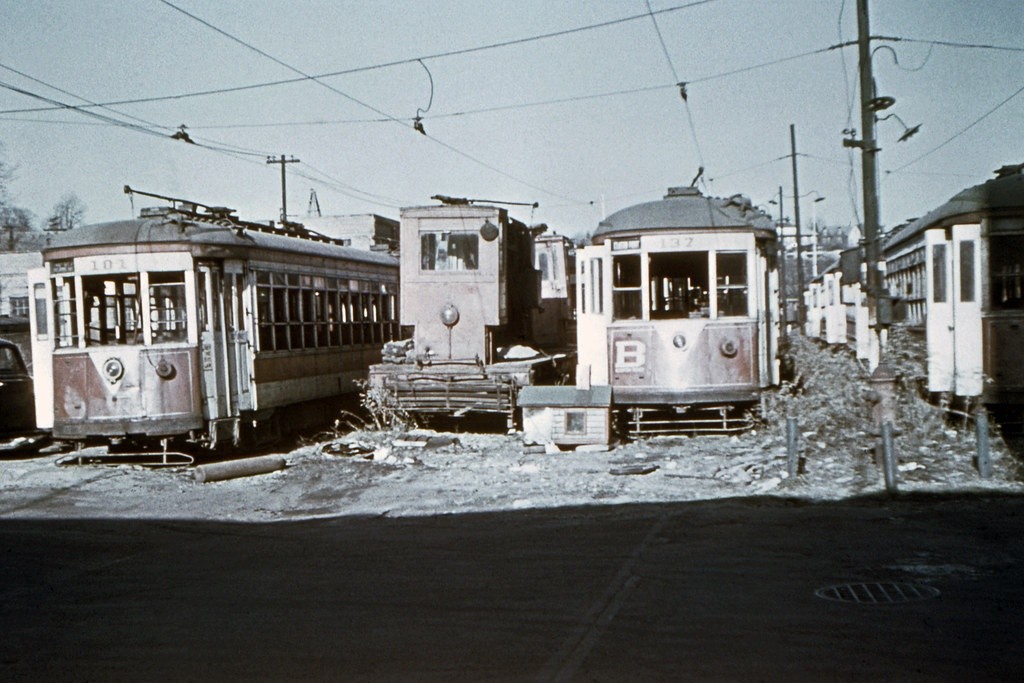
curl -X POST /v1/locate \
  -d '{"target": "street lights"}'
[861,111,923,376]
[793,190,826,323]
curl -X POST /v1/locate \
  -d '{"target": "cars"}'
[0,339,41,439]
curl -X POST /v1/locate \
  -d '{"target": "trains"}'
[30,185,405,448]
[801,164,1024,425]
[517,188,788,438]
[365,192,578,434]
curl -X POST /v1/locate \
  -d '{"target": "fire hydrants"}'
[859,359,903,470]
